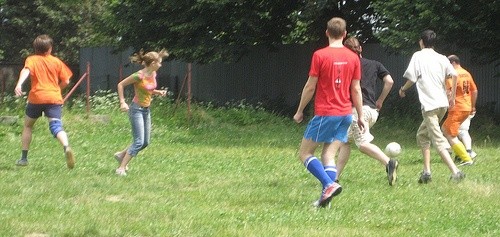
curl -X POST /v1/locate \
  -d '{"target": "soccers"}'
[385,141,402,158]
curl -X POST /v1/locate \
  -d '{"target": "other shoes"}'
[417,172,432,184]
[447,172,466,186]
[114,152,129,171]
[116,169,127,176]
[65,146,75,169]
[457,160,473,166]
[467,151,477,158]
[385,159,399,187]
[15,160,28,166]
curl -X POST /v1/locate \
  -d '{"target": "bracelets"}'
[400,86,405,92]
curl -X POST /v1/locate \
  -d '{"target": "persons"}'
[399,30,465,184]
[113,48,169,176]
[441,55,478,166]
[13,34,74,168]
[334,37,398,187]
[294,17,365,206]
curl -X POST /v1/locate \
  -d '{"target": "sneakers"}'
[309,181,342,209]
